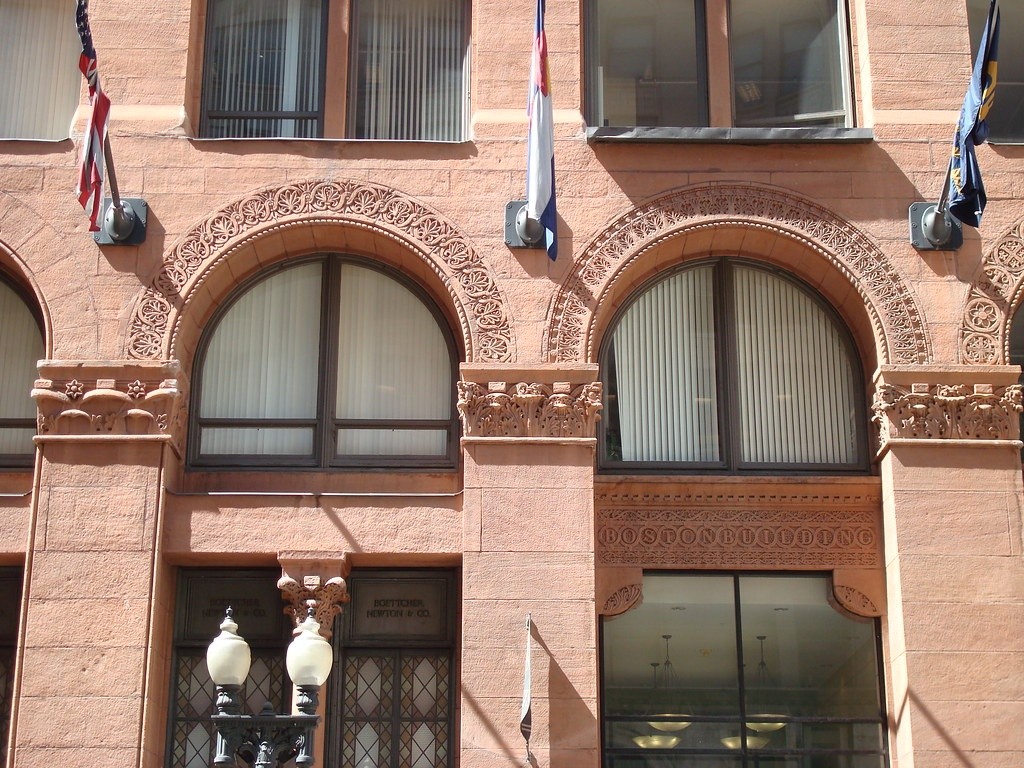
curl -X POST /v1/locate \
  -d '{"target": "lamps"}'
[721,664,769,749]
[745,636,792,734]
[647,635,695,732]
[632,663,683,749]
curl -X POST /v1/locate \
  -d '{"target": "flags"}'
[524,0,559,261]
[946,1,1002,229]
[66,0,114,241]
